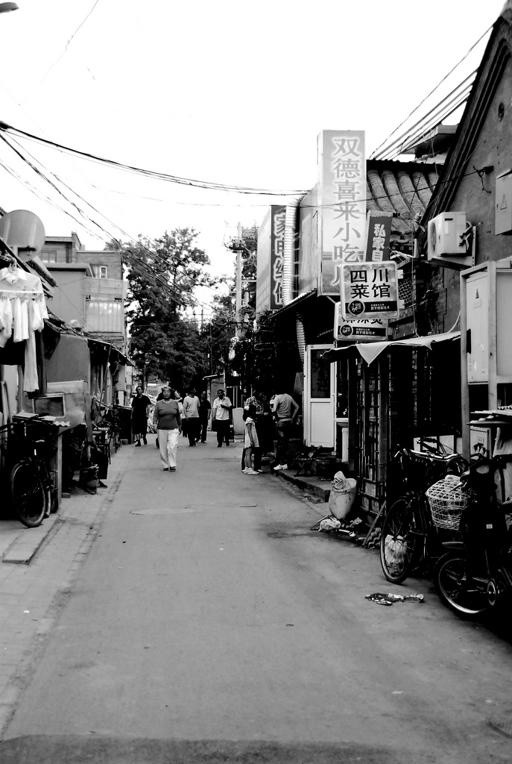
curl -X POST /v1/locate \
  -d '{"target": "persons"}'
[270,387,300,471]
[153,385,233,449]
[130,385,151,446]
[152,386,182,471]
[242,409,261,475]
[238,388,268,471]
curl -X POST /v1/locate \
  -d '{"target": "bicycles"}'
[0,412,52,528]
[378,438,469,585]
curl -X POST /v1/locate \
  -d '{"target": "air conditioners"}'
[427,210,476,268]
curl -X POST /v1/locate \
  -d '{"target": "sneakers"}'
[162,467,177,472]
[240,466,265,476]
[272,463,289,471]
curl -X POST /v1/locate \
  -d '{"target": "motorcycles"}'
[425,452,512,621]
